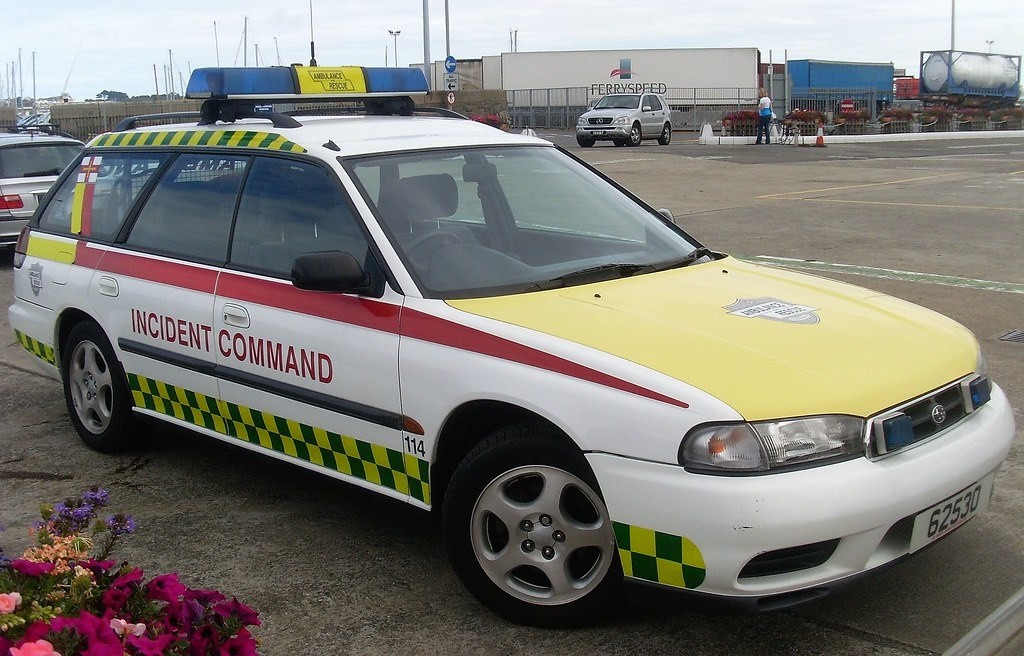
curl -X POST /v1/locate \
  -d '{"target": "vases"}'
[729,116,1024,137]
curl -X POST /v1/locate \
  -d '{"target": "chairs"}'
[255,182,347,274]
[385,173,479,263]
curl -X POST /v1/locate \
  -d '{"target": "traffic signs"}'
[443,74,459,91]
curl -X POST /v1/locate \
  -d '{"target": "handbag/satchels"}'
[771,111,776,119]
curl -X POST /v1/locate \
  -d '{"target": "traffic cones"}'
[812,121,828,147]
[721,119,728,136]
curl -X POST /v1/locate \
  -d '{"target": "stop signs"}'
[841,100,855,114]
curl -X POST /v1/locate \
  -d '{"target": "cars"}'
[5,62,1017,637]
[575,92,673,148]
[16,111,52,134]
[0,125,87,248]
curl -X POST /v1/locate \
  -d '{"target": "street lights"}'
[986,40,994,54]
[388,29,401,68]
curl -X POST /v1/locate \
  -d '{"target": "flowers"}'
[881,107,912,119]
[836,110,869,121]
[923,107,951,120]
[470,114,500,125]
[724,110,759,120]
[785,110,827,120]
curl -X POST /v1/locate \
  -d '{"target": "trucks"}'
[761,49,1022,108]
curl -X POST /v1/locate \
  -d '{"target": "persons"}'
[754,88,772,145]
